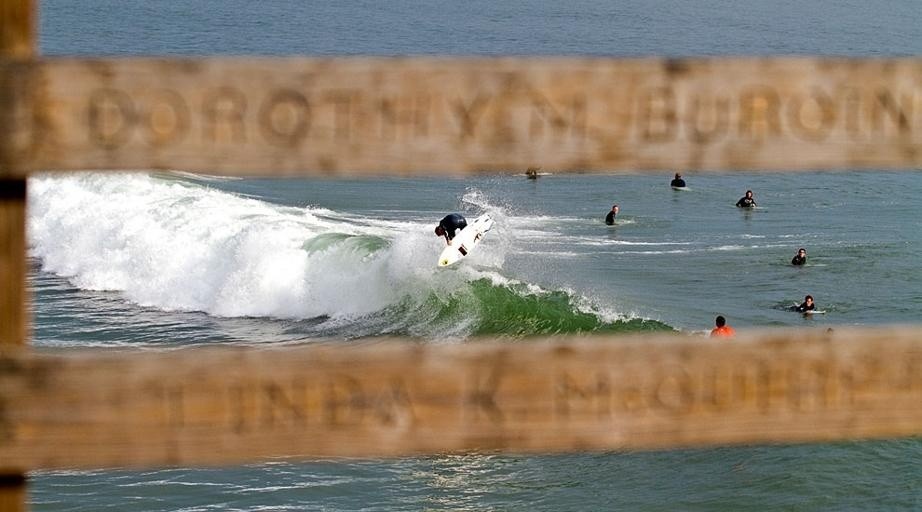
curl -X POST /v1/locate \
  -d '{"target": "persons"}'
[734,189,757,210]
[604,203,623,225]
[795,293,826,315]
[709,313,735,336]
[791,247,807,266]
[435,211,467,247]
[669,171,686,190]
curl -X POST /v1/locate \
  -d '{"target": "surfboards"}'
[436,210,495,266]
[806,311,826,314]
